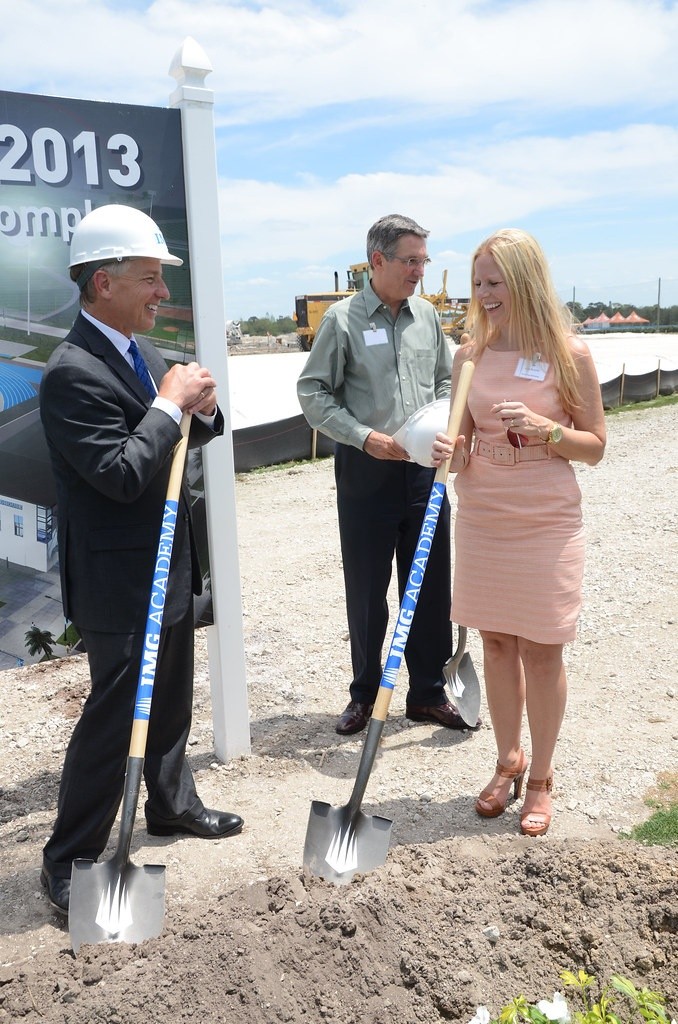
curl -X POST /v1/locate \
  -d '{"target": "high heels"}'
[475,749,528,817]
[520,772,553,836]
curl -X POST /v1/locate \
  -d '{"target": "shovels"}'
[68,406,197,952]
[304,360,477,887]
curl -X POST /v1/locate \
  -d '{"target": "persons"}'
[39,201,245,918]
[426,226,609,836]
[296,213,484,736]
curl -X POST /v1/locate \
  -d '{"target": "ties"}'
[128,340,158,399]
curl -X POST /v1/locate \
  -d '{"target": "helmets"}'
[68,203,183,267]
[392,396,451,469]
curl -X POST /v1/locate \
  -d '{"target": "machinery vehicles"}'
[291,261,471,349]
[226,319,247,343]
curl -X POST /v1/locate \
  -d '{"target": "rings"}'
[199,392,206,399]
[509,419,514,426]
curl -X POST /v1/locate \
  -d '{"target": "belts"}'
[473,439,561,466]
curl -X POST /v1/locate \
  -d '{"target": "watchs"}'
[538,420,563,447]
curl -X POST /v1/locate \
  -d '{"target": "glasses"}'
[500,398,529,450]
[379,249,431,268]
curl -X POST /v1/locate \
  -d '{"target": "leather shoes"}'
[335,695,378,735]
[405,697,482,729]
[146,798,243,839]
[40,862,72,915]
